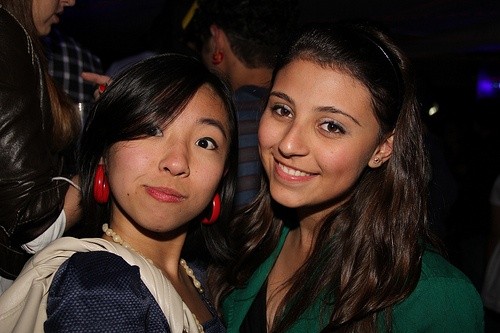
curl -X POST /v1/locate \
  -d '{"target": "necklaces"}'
[101,223,224,333]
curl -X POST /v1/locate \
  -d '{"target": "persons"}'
[213,20,485,333]
[0,0,289,308]
[43,53,240,333]
